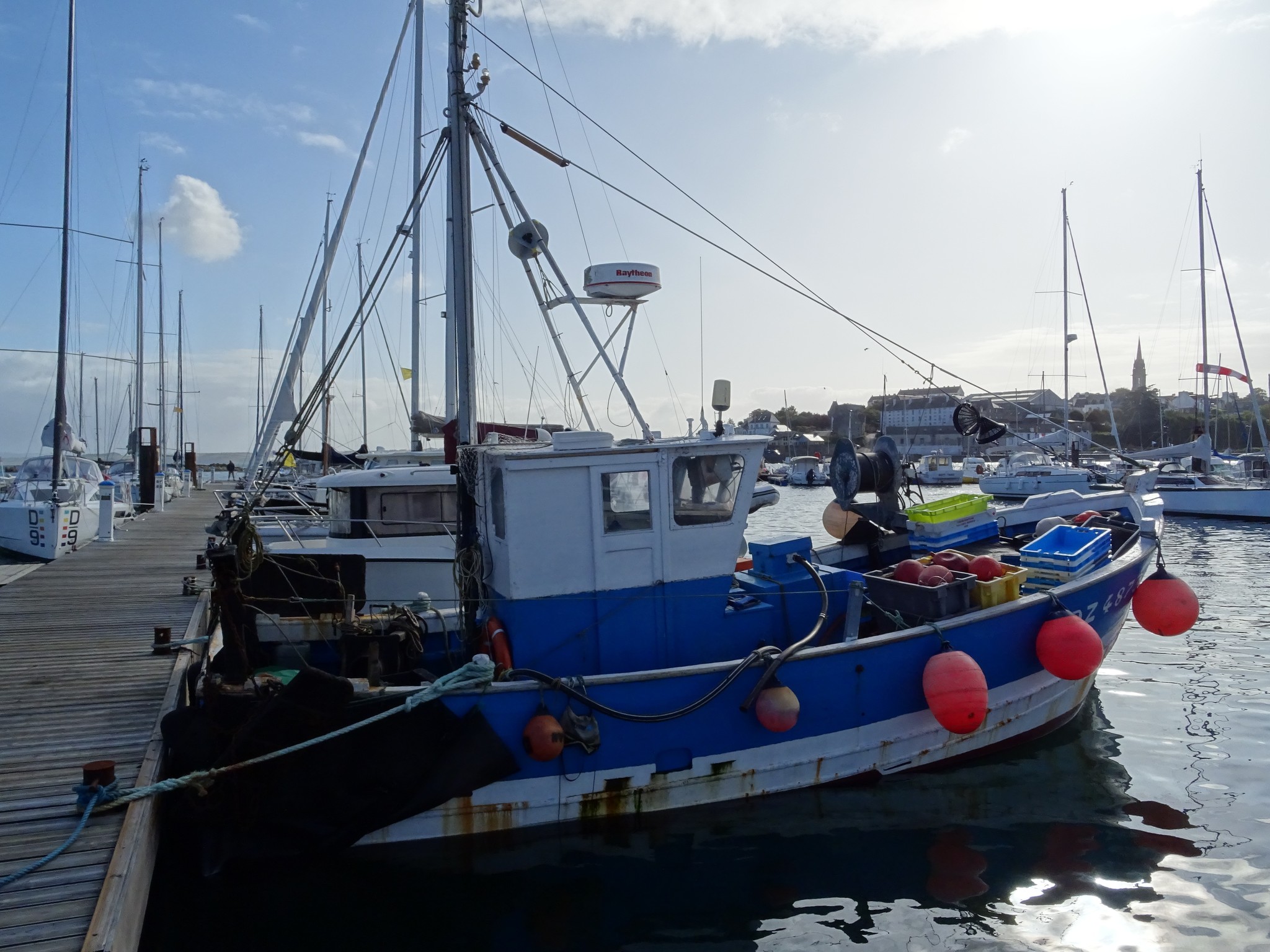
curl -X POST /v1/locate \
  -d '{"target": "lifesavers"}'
[102,473,111,481]
[480,615,514,685]
[815,452,820,458]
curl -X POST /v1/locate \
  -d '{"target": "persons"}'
[227,460,236,481]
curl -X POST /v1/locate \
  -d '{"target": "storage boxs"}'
[904,494,1001,558]
[1018,525,1112,596]
[1081,515,1141,559]
[863,549,1028,632]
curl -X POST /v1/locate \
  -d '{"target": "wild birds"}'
[824,387,826,389]
[864,348,870,351]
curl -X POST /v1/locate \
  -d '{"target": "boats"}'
[755,376,990,486]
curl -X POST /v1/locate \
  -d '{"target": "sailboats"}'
[2,0,199,563]
[206,0,1165,899]
[976,156,1270,520]
